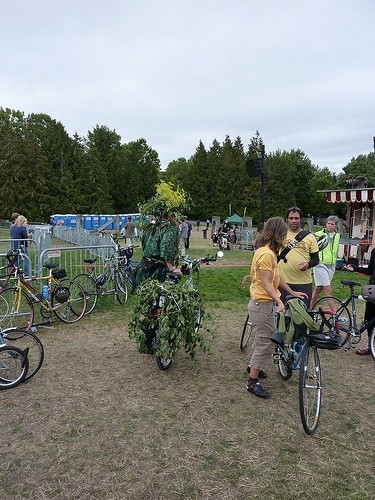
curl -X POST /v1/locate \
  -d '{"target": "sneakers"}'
[338,316,348,324]
[246,382,270,397]
[246,365,268,378]
[356,346,372,355]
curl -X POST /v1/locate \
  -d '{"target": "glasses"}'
[288,207,301,213]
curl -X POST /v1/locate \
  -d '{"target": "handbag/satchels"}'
[120,247,134,261]
[53,268,66,279]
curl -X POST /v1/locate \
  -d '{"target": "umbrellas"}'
[223,214,242,224]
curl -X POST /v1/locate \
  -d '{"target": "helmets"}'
[317,236,328,250]
[306,333,342,350]
[55,287,70,304]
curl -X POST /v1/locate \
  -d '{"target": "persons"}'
[137,204,182,356]
[179,218,193,257]
[245,218,308,398]
[196,219,239,245]
[51,218,62,235]
[309,215,349,325]
[273,206,319,356]
[345,248,375,354]
[10,213,32,276]
[121,216,139,252]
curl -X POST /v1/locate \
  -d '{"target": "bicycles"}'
[0,245,141,391]
[238,275,253,352]
[309,279,375,362]
[144,251,224,369]
[272,294,323,436]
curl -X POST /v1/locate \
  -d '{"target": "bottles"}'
[43,284,49,298]
